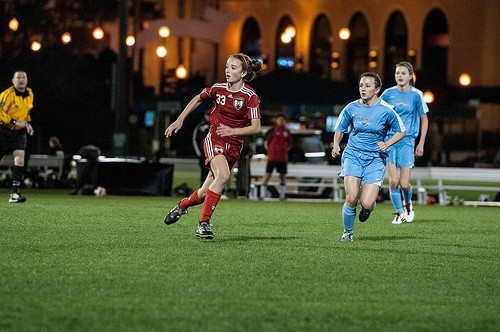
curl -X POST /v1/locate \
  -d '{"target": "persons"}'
[377,62,429,224]
[331,73,406,243]
[163,54,261,239]
[259,116,294,201]
[193,111,227,199]
[0,71,34,203]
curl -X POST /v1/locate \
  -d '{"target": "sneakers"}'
[8,193,27,203]
[392,212,406,225]
[402,201,414,223]
[341,232,354,242]
[164,197,189,224]
[359,209,371,222]
[196,221,215,239]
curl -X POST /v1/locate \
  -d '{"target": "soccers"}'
[94,185,107,196]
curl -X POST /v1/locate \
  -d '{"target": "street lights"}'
[125,26,187,157]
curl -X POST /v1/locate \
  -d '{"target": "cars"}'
[287,130,331,166]
